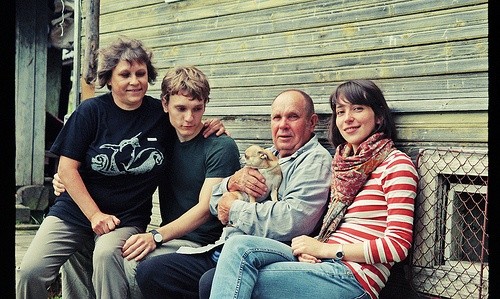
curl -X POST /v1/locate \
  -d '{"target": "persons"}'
[209,79,419,298]
[135,88,334,299]
[14,36,232,299]
[51,61,243,299]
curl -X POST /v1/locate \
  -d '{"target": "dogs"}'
[218,145,284,227]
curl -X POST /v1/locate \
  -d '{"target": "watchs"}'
[334,244,345,262]
[149,230,163,249]
